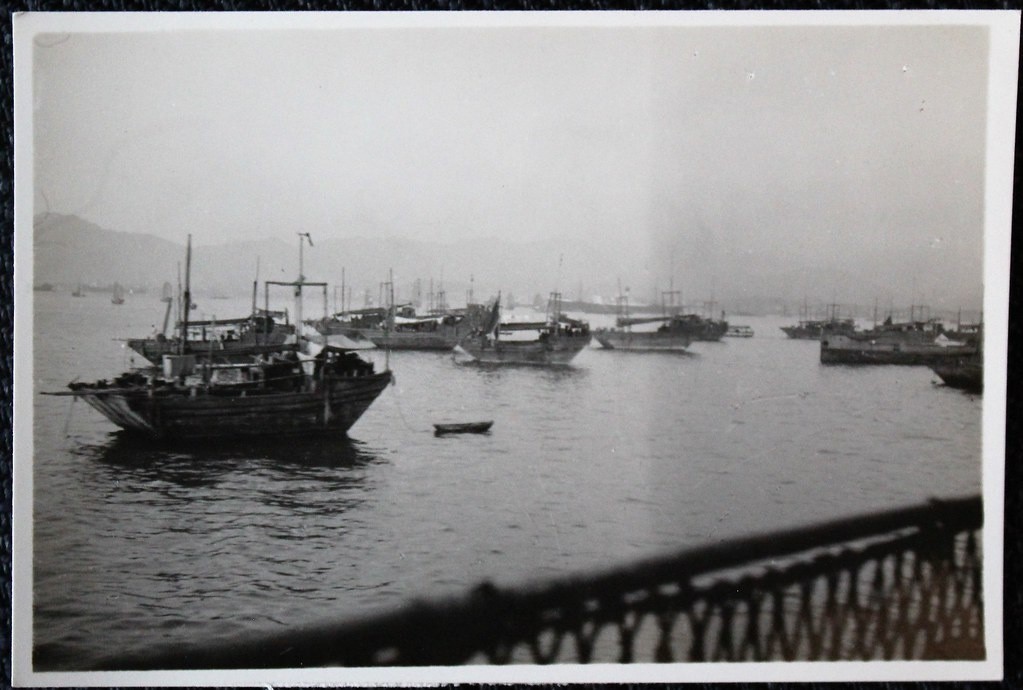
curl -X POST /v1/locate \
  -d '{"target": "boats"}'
[302,268,492,351]
[594,280,692,351]
[725,325,755,337]
[780,296,856,339]
[819,304,983,366]
[436,420,492,433]
[657,291,729,341]
[460,292,591,364]
[933,352,983,389]
[69,230,393,433]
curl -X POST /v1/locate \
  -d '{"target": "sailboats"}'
[110,280,123,305]
[72,280,84,296]
[163,281,173,300]
